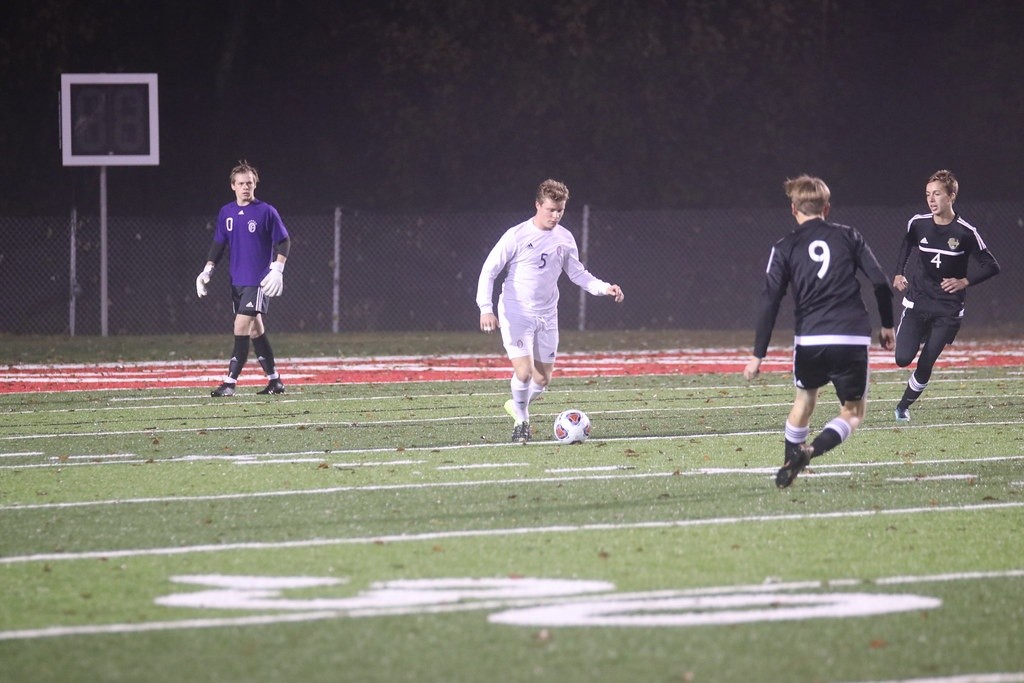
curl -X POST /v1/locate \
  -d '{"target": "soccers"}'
[551,409,593,446]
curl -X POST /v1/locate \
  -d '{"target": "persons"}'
[744,177,897,489]
[475,177,625,443]
[892,169,1002,420]
[195,157,290,396]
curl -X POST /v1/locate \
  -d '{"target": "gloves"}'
[196,264,216,298]
[260,261,284,297]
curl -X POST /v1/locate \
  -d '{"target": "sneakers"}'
[512,421,529,445]
[895,405,910,422]
[257,374,284,394]
[504,399,533,439]
[776,444,815,489]
[211,382,235,397]
